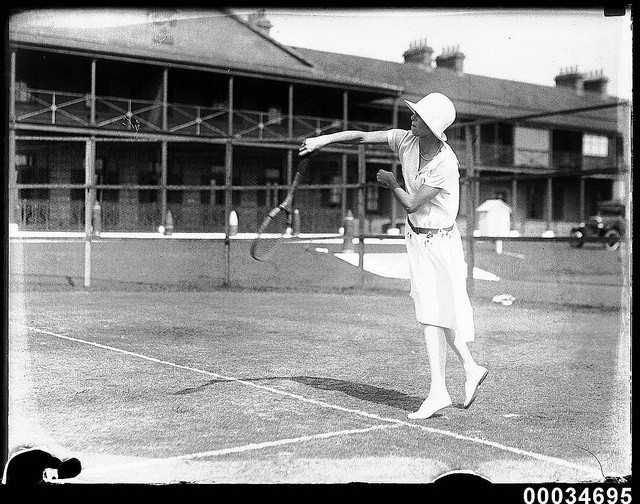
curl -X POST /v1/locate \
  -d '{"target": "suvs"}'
[571,200,623,251]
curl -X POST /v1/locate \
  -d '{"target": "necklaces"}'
[419,141,442,161]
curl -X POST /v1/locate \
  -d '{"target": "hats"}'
[404,91,456,141]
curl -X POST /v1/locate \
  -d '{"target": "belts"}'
[408,217,453,235]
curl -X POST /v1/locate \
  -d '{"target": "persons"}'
[297,92,489,420]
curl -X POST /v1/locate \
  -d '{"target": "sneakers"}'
[463,366,489,408]
[407,391,452,420]
